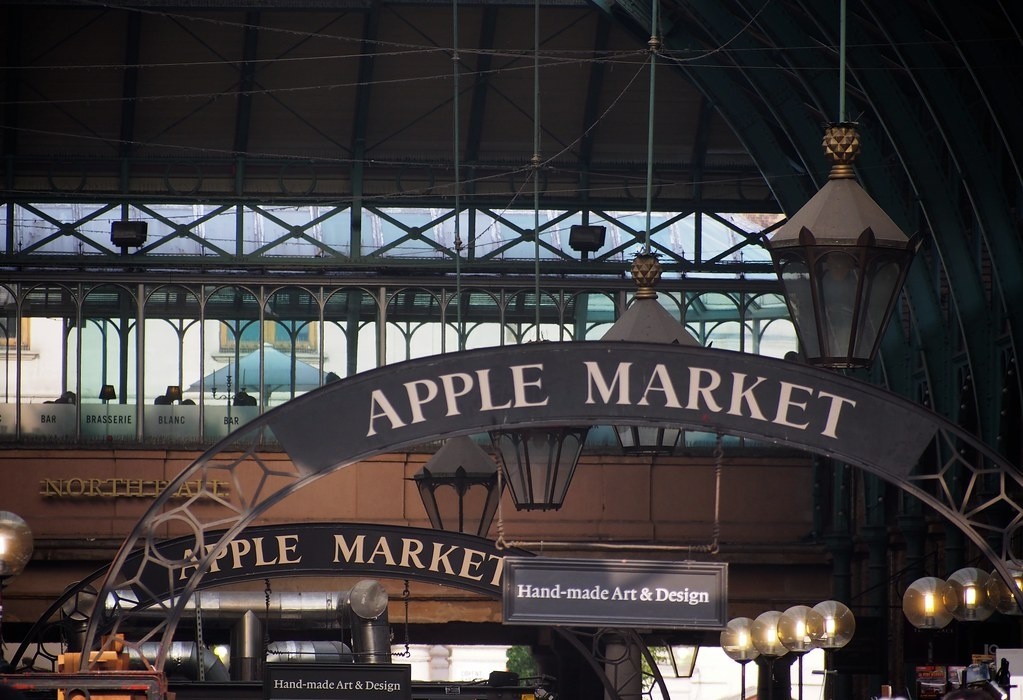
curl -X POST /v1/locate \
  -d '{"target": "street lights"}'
[902,560,1023,699]
[0,510,37,700]
[720,599,856,700]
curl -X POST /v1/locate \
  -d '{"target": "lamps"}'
[0,510,33,594]
[413,0,507,542]
[903,577,953,665]
[941,567,995,621]
[778,605,817,700]
[719,617,760,700]
[481,0,598,510]
[756,0,924,375]
[805,600,855,700]
[602,0,704,349]
[750,611,791,700]
[985,558,1023,614]
